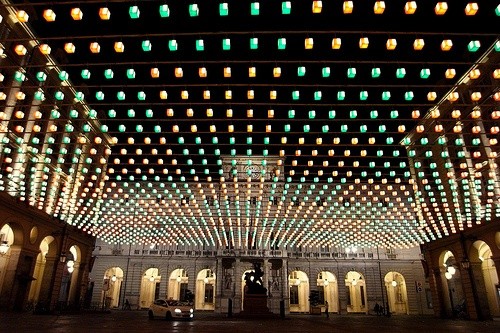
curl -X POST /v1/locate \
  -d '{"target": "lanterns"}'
[0,0,500,251]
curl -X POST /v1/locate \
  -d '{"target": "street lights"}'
[44,252,67,315]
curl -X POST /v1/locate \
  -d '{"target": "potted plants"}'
[308,290,321,315]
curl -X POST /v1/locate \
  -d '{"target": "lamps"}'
[0,1,500,286]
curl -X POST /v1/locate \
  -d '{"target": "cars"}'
[148,299,194,320]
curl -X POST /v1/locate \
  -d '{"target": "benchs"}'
[365,309,383,315]
[124,304,140,310]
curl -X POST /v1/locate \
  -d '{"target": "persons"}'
[325,301,329,321]
[379,305,383,316]
[373,302,379,316]
[123,298,131,310]
